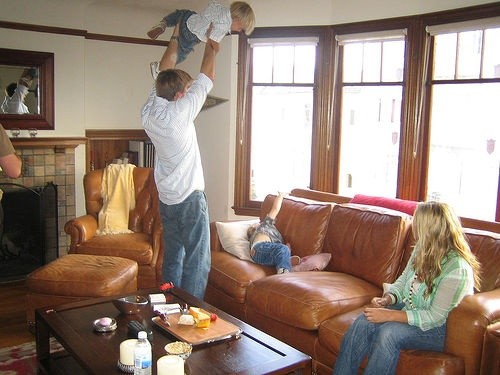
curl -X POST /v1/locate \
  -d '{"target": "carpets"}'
[0,337,90,375]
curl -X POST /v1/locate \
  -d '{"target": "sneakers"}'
[147,22,166,40]
[150,61,161,81]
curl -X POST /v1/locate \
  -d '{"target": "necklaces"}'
[409,272,417,309]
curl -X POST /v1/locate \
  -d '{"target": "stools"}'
[27,255,138,333]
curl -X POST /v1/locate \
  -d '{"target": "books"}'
[129,140,155,168]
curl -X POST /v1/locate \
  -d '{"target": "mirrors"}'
[0,47,56,130]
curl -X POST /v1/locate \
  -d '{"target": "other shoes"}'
[287,255,300,265]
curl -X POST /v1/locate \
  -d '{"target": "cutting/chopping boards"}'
[152,312,240,345]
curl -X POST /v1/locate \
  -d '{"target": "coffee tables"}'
[34,285,312,375]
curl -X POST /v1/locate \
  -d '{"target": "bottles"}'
[134,331,152,375]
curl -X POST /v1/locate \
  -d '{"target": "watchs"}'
[171,36,179,40]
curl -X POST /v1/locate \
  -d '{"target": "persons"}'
[248,190,292,274]
[2,67,36,113]
[0,123,22,235]
[146,1,255,81]
[331,202,484,375]
[142,11,215,300]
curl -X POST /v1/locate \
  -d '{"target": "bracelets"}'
[383,292,396,306]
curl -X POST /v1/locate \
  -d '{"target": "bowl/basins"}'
[164,342,192,360]
[112,295,148,314]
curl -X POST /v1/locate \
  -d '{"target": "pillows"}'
[289,253,331,271]
[215,219,262,263]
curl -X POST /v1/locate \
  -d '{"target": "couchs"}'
[204,189,500,375]
[64,167,164,290]
